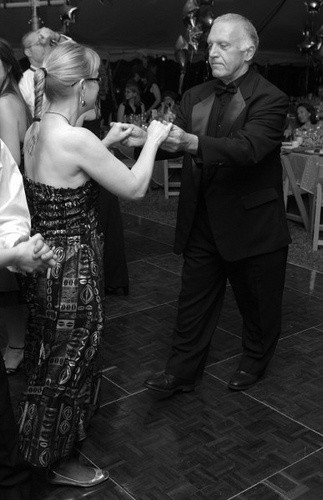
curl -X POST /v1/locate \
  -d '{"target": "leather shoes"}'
[228,367,265,390]
[145,373,197,393]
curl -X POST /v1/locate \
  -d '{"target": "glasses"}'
[71,76,104,87]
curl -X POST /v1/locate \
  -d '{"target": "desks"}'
[103,127,182,199]
[280,148,323,228]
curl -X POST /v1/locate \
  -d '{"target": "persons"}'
[293,104,317,141]
[109,13,289,395]
[101,53,189,123]
[16,29,99,122]
[0,140,55,499]
[0,38,32,168]
[21,43,173,486]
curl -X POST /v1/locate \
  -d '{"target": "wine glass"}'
[120,114,147,127]
[290,125,323,152]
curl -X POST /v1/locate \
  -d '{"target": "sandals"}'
[49,467,110,488]
